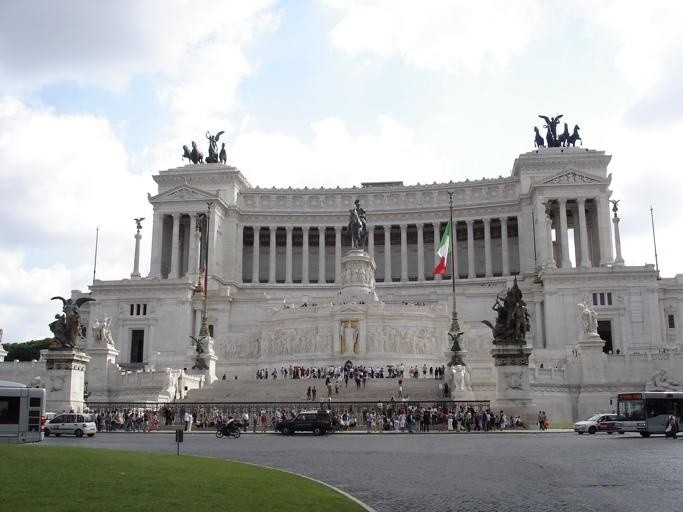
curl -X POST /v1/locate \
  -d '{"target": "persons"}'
[193,338,204,356]
[48,295,115,351]
[546,117,560,139]
[576,301,598,335]
[345,199,368,235]
[339,321,359,356]
[480,275,531,344]
[205,128,226,157]
[254,364,450,400]
[447,331,465,351]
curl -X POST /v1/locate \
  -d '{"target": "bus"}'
[610,390,683,438]
[0,380,47,443]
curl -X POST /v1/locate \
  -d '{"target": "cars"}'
[574,413,625,434]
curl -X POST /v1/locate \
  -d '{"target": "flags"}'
[432,216,454,275]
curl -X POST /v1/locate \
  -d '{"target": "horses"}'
[567,124,582,147]
[182,141,203,164]
[349,207,369,250]
[558,123,571,147]
[219,143,227,164]
[546,124,555,147]
[533,126,546,148]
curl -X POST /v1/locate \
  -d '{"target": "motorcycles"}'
[216,415,242,439]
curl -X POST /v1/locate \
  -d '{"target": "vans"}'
[44,413,97,437]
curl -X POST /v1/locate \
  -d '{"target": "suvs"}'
[276,409,334,436]
